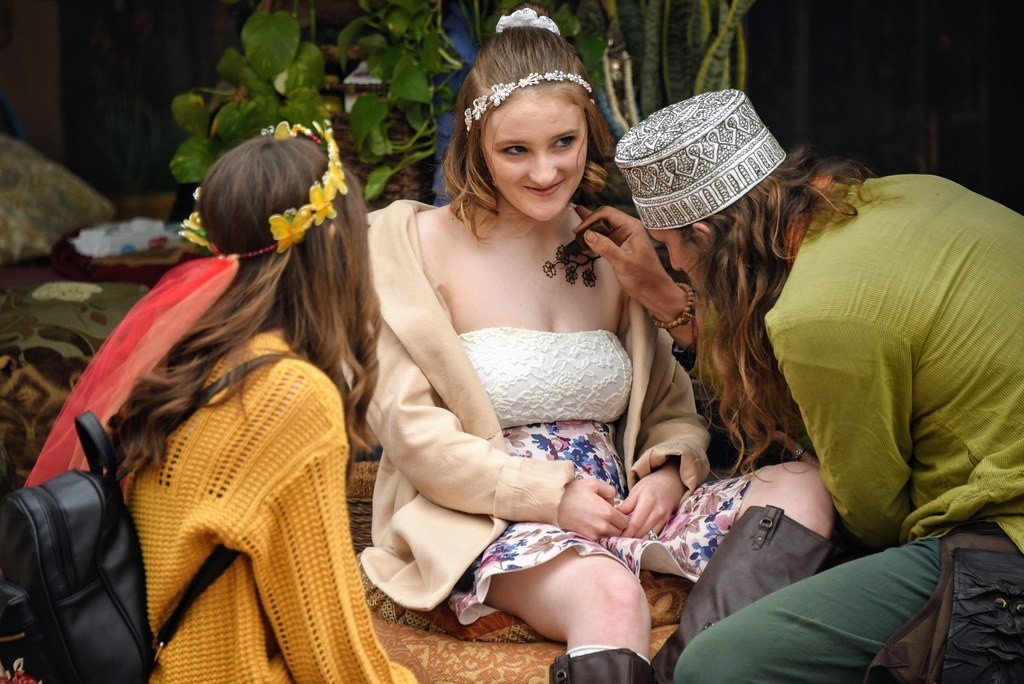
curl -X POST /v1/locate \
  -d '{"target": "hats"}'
[613,89,787,230]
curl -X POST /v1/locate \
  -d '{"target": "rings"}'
[647,530,657,540]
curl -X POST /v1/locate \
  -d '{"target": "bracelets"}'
[651,282,694,328]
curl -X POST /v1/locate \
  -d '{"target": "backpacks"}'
[2,357,299,683]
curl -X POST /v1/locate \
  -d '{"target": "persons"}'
[72,130,418,684]
[340,7,833,684]
[572,88,1023,684]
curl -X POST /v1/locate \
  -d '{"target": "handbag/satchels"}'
[864,521,1023,683]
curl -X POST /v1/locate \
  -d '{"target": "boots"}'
[549,648,654,684]
[651,505,835,684]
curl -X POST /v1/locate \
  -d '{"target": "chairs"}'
[346,460,695,684]
